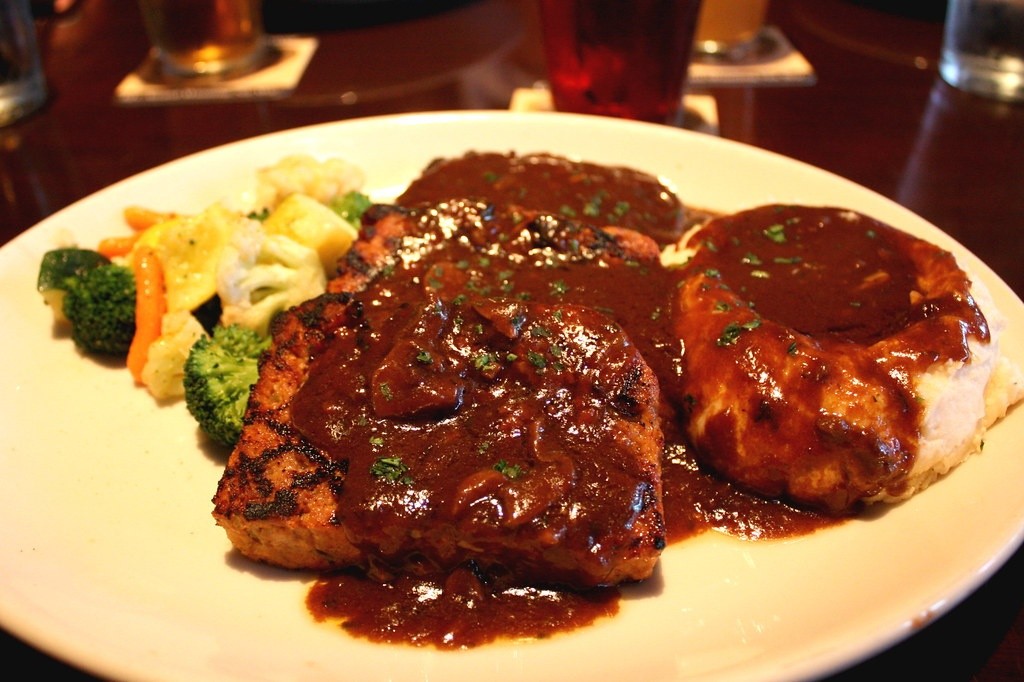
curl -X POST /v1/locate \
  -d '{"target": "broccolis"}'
[59,152,372,446]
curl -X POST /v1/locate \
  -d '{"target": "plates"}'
[0,109,1024,682]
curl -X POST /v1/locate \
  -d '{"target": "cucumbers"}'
[39,248,113,322]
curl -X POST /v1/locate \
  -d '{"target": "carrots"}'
[98,234,140,259]
[126,244,166,380]
[124,204,173,230]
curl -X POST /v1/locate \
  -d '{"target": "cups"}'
[692,0,771,67]
[936,0,1024,104]
[0,0,48,129]
[532,0,703,130]
[138,0,281,88]
